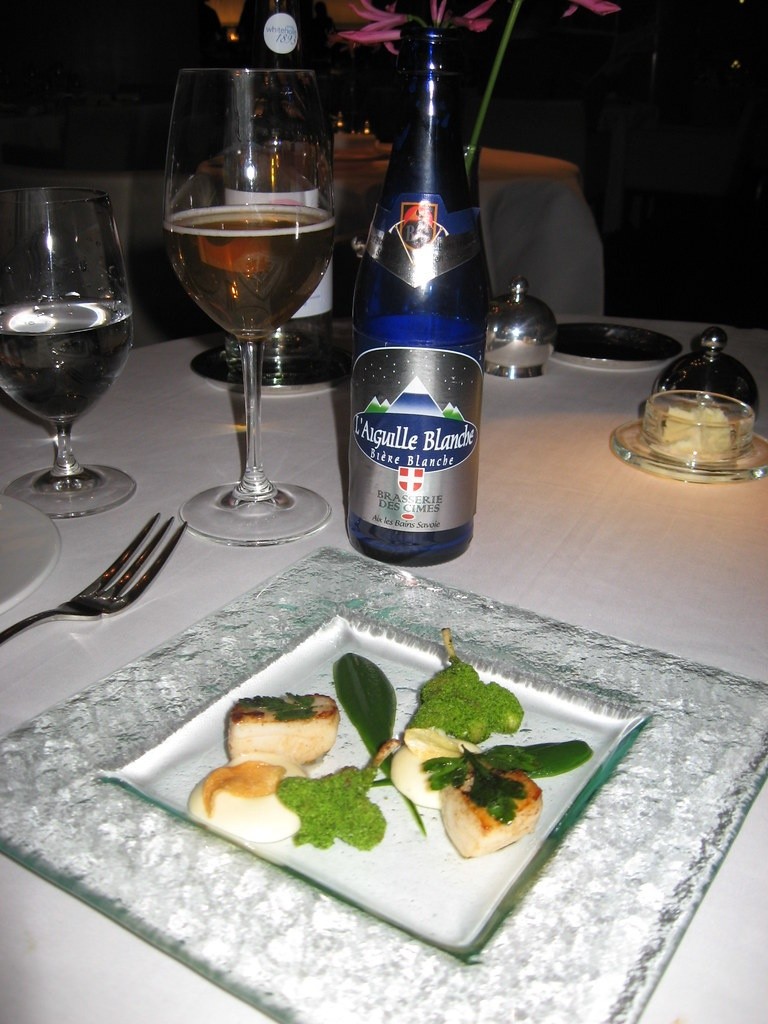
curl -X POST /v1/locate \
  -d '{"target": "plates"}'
[550,322,683,369]
[189,344,351,389]
[0,494,61,616]
[333,152,388,159]
[0,544,768,1024]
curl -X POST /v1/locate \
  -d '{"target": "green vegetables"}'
[420,743,545,828]
[237,691,321,723]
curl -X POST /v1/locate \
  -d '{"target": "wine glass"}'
[0,186,138,520]
[163,68,336,549]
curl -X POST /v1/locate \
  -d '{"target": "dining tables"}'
[0,316,768,1024]
[0,140,605,349]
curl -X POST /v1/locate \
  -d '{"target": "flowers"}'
[337,0,622,178]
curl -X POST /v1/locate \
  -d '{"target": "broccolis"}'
[406,655,524,743]
[274,766,386,850]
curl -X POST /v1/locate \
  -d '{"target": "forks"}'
[0,513,187,648]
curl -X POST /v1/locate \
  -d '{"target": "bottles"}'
[223,0,338,388]
[347,26,492,567]
